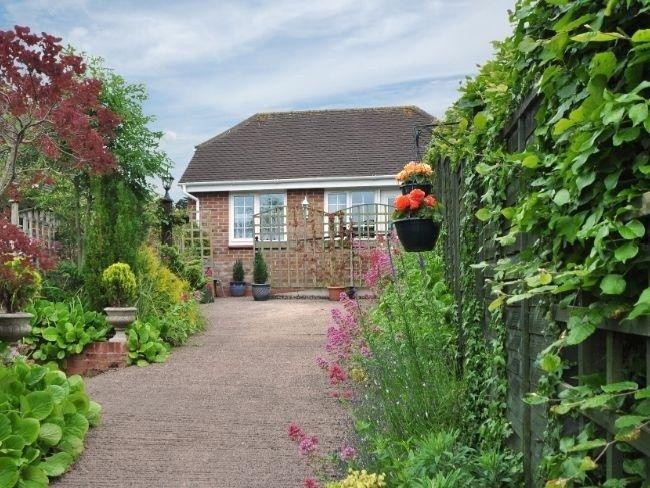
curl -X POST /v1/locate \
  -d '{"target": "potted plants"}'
[251,251,271,301]
[102,262,137,342]
[0,256,43,366]
[230,259,246,296]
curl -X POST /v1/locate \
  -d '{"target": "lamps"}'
[301,196,309,219]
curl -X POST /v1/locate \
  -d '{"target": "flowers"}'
[391,189,445,222]
[396,161,438,183]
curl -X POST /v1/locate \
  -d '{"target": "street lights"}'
[158,165,176,265]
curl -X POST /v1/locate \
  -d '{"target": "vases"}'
[327,287,345,301]
[400,183,433,197]
[392,218,442,252]
